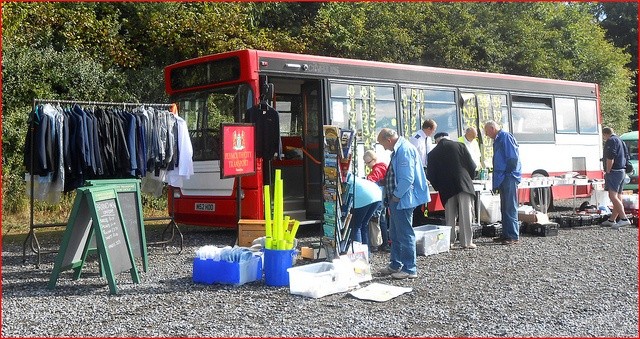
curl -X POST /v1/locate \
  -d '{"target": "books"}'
[320,125,354,262]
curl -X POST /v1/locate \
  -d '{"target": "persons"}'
[348,174,382,263]
[363,150,388,253]
[408,119,437,180]
[426,132,477,250]
[377,128,432,279]
[602,126,635,229]
[485,119,522,245]
[458,126,483,180]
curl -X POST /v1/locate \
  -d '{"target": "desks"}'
[532,178,605,213]
[466,175,552,225]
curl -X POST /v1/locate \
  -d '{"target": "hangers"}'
[257,94,272,110]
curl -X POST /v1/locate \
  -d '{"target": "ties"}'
[425,138,428,165]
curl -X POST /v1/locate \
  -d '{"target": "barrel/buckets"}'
[262,245,297,287]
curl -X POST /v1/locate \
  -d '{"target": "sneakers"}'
[392,271,417,279]
[602,221,616,226]
[462,242,476,249]
[612,219,631,229]
[502,238,519,244]
[450,243,454,247]
[380,266,401,275]
[493,236,506,242]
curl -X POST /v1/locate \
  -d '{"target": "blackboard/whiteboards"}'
[89,187,140,295]
[92,180,148,277]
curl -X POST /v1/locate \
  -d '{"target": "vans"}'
[618,131,639,192]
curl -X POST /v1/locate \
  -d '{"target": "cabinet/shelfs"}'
[238,218,293,247]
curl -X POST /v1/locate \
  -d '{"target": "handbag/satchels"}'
[621,140,632,173]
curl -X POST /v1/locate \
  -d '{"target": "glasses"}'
[364,158,373,163]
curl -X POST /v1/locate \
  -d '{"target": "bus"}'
[163,48,607,232]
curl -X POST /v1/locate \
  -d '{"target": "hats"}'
[434,132,448,142]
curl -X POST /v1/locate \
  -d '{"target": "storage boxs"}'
[298,244,326,260]
[413,224,452,257]
[529,222,558,237]
[192,256,261,285]
[481,222,504,237]
[286,261,349,299]
[556,205,638,228]
[517,214,547,233]
